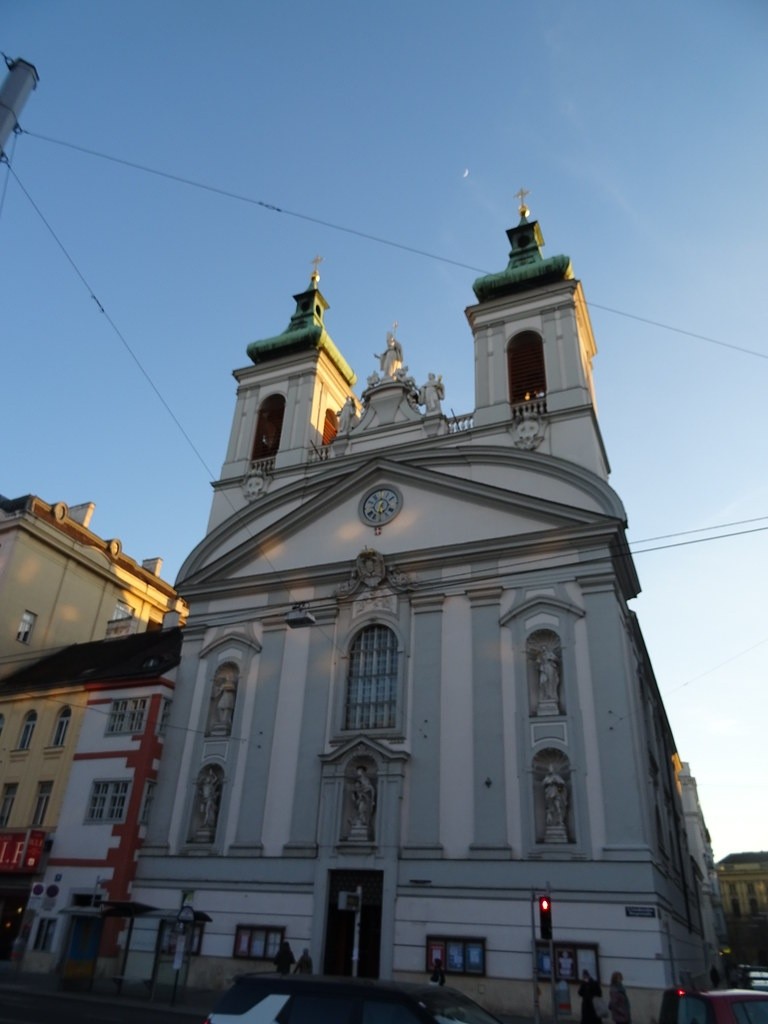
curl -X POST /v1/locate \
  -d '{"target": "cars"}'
[748,971,768,990]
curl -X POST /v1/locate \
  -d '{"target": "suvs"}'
[203,974,505,1024]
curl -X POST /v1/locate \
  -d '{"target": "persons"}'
[416,373,445,418]
[198,768,220,828]
[375,332,402,382]
[272,943,295,976]
[346,765,376,827]
[608,973,632,1024]
[524,390,546,415]
[540,763,568,827]
[212,671,236,723]
[429,959,445,986]
[739,966,754,989]
[291,949,313,976]
[710,964,720,988]
[577,970,603,1024]
[334,396,357,434]
[729,964,742,988]
[534,645,560,700]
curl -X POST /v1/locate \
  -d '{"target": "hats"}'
[303,949,308,952]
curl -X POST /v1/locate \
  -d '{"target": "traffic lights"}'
[539,894,554,941]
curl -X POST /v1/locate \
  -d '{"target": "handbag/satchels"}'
[594,998,607,1018]
[429,980,440,987]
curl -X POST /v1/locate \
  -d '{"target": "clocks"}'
[363,489,399,522]
